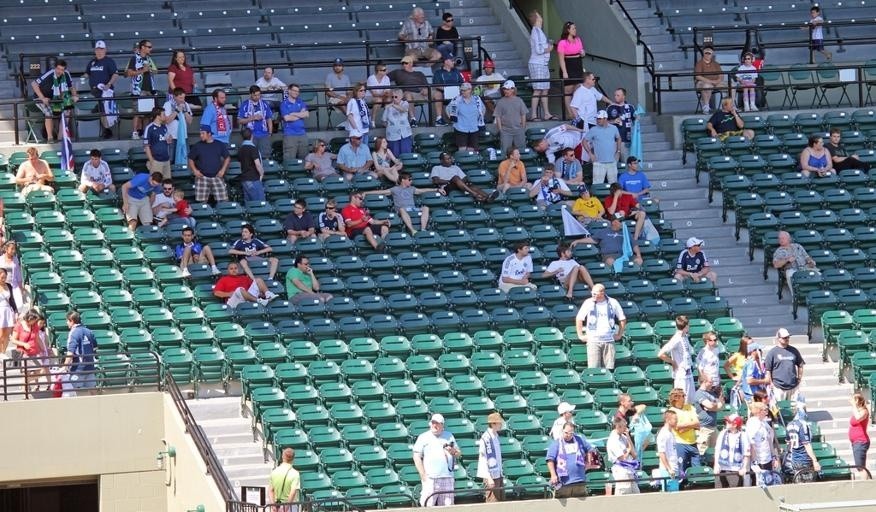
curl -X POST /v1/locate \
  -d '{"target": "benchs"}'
[651,2,876,57]
[0,0,479,58]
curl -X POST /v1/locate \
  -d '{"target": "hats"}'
[724,414,743,427]
[95,40,107,49]
[776,328,792,339]
[686,237,702,248]
[747,342,765,353]
[579,184,589,193]
[502,80,515,89]
[200,125,214,135]
[488,413,503,423]
[334,58,343,65]
[349,129,364,137]
[431,414,444,425]
[628,157,641,163]
[460,83,472,91]
[401,56,413,64]
[703,48,713,54]
[558,402,576,414]
[611,212,624,221]
[483,60,494,70]
[594,110,609,119]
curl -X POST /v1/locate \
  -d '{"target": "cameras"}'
[443,442,454,448]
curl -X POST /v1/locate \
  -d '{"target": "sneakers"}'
[411,119,418,128]
[212,269,222,276]
[259,298,271,307]
[266,294,279,302]
[434,118,448,127]
[182,270,192,277]
[487,191,499,203]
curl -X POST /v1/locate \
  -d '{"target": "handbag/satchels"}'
[10,349,24,367]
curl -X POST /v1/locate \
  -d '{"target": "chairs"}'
[680,109,874,436]
[0,121,851,506]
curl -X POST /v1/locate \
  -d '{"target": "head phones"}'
[742,52,755,63]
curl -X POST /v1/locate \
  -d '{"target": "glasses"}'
[446,19,454,22]
[164,188,171,190]
[352,137,362,140]
[325,207,335,210]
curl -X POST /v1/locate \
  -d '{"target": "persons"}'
[61,312,96,394]
[673,237,717,283]
[529,9,586,121]
[693,47,765,141]
[809,7,832,64]
[848,393,870,472]
[13,38,662,306]
[413,413,461,507]
[546,402,594,497]
[268,448,301,510]
[574,284,626,372]
[477,413,506,502]
[799,136,835,177]
[607,315,822,495]
[773,231,821,303]
[397,9,460,67]
[823,129,873,173]
[1,240,52,392]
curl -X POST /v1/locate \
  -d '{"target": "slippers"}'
[544,116,559,121]
[528,118,542,122]
